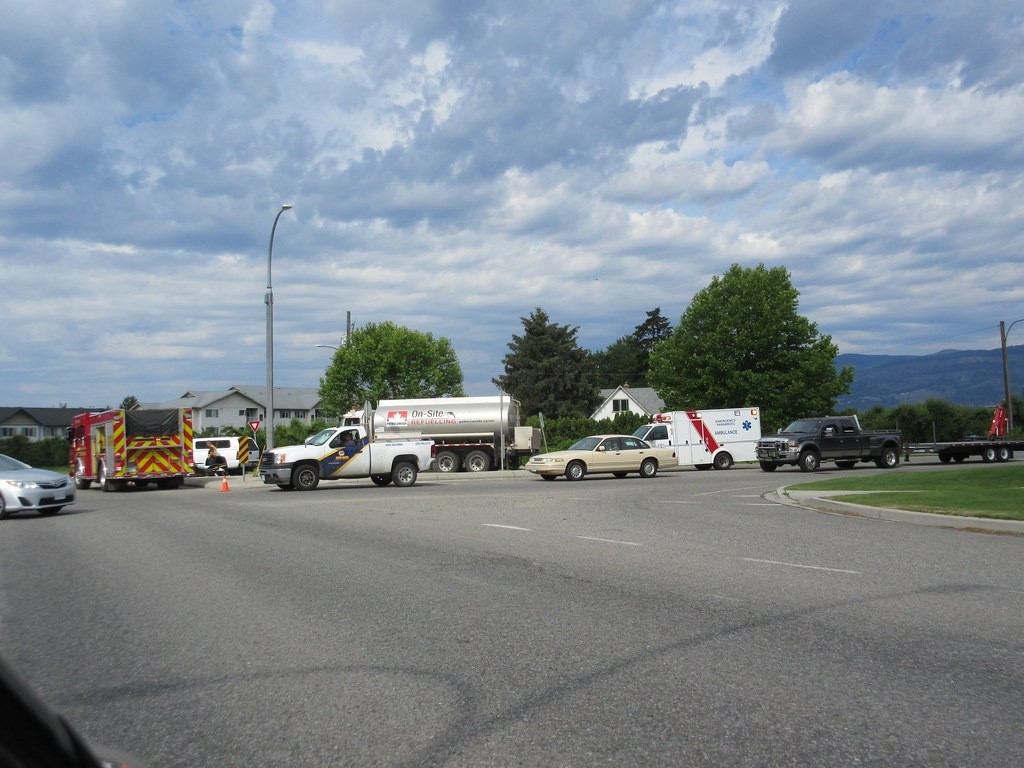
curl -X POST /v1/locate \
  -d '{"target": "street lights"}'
[265,204,293,449]
[1004,419,1009,433]
[995,426,999,436]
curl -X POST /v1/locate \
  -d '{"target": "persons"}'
[606,440,619,450]
[205,441,217,477]
[342,433,354,447]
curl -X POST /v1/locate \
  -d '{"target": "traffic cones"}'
[221,476,230,493]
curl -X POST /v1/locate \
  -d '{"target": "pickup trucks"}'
[258,423,437,489]
[754,414,902,472]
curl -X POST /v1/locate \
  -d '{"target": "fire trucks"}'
[66,407,193,491]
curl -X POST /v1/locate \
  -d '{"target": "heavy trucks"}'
[305,394,542,473]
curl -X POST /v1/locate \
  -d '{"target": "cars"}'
[0,454,78,518]
[524,433,679,481]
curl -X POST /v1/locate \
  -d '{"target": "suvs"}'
[192,436,259,471]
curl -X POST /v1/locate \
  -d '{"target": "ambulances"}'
[611,407,761,470]
